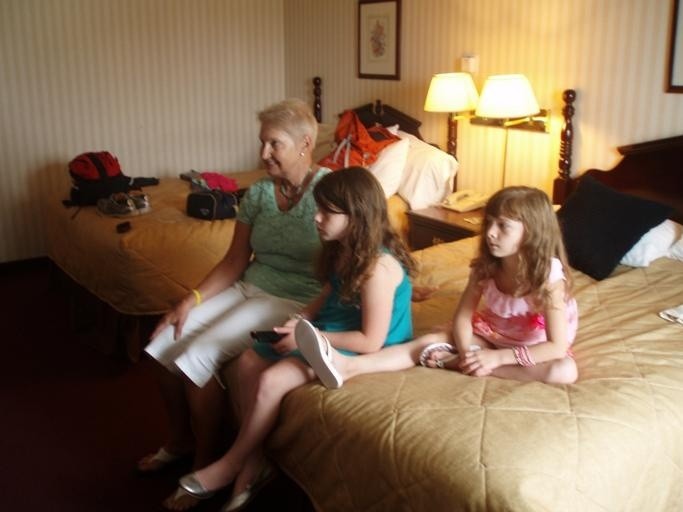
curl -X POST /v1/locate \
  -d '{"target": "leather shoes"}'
[97,191,138,218]
[128,189,152,214]
[223,461,275,512]
[177,454,240,498]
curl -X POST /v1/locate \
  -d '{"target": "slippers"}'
[133,444,183,473]
[160,486,200,512]
[417,342,482,370]
[292,318,342,391]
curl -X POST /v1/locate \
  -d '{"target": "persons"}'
[294,187,579,391]
[131,99,347,511]
[178,167,413,511]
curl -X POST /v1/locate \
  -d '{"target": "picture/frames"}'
[663,0,683,93]
[355,0,404,81]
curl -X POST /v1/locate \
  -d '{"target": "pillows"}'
[397,130,461,212]
[556,172,673,283]
[618,219,682,271]
[361,124,411,202]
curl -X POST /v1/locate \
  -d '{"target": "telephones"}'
[428,189,492,213]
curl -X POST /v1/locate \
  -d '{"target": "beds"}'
[224,89,683,512]
[42,77,440,364]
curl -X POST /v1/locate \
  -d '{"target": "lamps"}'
[473,72,541,191]
[422,72,480,194]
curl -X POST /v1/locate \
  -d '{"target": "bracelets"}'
[513,345,534,368]
[191,289,202,305]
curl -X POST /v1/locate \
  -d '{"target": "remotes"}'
[251,330,287,344]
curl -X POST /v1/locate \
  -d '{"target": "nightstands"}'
[404,207,488,251]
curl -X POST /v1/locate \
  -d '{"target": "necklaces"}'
[277,168,315,202]
